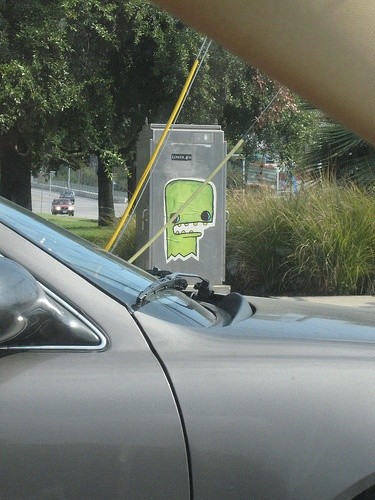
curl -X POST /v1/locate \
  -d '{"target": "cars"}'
[0,197,374,496]
[50,198,73,216]
[60,191,74,202]
